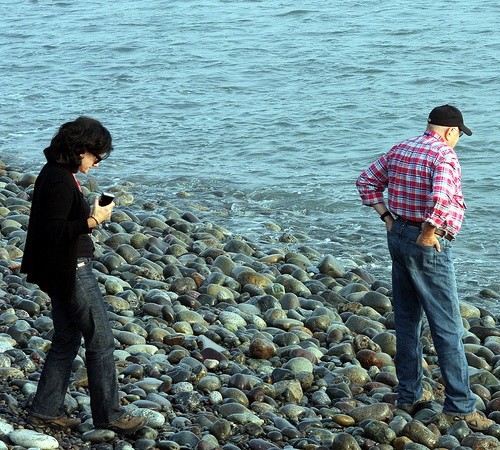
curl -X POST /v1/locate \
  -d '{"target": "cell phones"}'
[99,192,115,207]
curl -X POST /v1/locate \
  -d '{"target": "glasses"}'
[453,128,464,138]
[93,154,103,164]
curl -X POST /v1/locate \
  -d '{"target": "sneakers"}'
[445,410,496,430]
[397,390,436,411]
[27,410,81,429]
[94,411,146,435]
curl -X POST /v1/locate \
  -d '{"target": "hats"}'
[427,104,472,136]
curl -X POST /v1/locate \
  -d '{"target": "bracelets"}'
[379,211,390,222]
[90,216,99,225]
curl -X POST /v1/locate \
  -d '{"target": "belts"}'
[397,216,456,242]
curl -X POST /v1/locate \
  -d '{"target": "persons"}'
[356,105,496,433]
[19,114,144,435]
